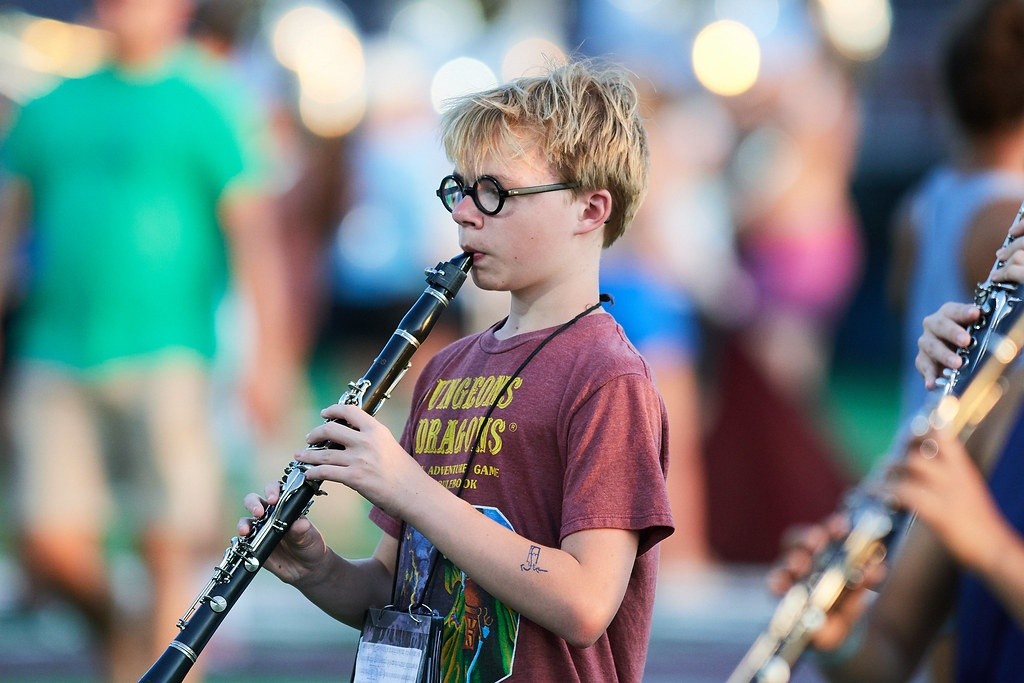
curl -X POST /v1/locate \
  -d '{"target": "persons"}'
[904,195,1024,514]
[194,21,321,488]
[748,48,862,416]
[919,4,1024,600]
[395,21,475,425]
[583,72,723,599]
[236,38,673,683]
[2,95,48,386]
[755,190,1024,683]
[5,0,306,682]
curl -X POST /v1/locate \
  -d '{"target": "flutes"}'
[727,304,1024,683]
[136,249,473,683]
[868,197,1024,591]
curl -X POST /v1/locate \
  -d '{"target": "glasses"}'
[435,174,579,216]
